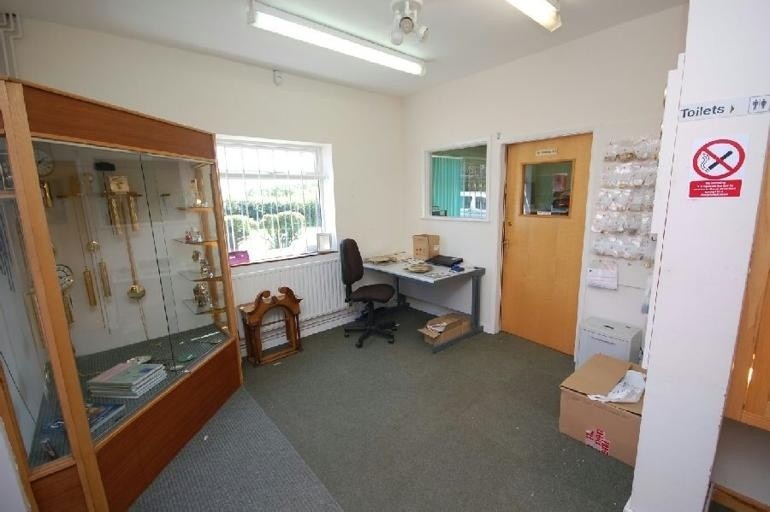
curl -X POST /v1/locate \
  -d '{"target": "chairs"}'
[340,238,397,348]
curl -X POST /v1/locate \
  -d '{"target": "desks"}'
[361,259,486,355]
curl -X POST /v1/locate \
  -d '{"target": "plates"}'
[407,265,433,273]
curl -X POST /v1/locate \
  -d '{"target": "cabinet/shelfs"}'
[171,206,226,317]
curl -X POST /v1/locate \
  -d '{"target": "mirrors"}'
[420,137,490,224]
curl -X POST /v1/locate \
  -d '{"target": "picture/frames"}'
[316,232,332,253]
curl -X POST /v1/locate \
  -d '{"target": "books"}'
[40,396,125,437]
[87,358,169,401]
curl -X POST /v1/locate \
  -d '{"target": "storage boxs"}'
[413,234,439,262]
[560,353,648,468]
[417,310,473,346]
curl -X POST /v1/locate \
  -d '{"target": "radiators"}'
[230,256,349,341]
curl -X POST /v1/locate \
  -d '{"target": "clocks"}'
[32,147,56,178]
